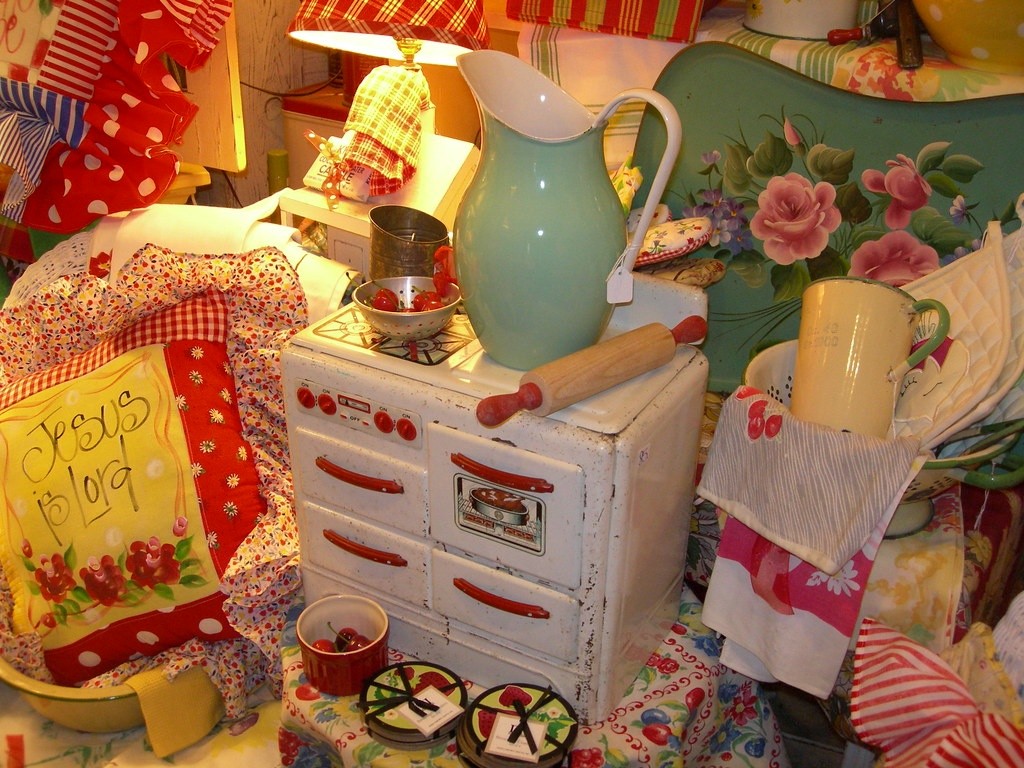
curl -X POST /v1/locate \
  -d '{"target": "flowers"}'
[673,108,1017,354]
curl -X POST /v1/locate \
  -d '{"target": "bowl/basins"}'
[0,657,142,735]
[913,0,1024,74]
[296,594,389,696]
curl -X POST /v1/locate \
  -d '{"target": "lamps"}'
[281,1,491,276]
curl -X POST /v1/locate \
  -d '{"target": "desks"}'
[280,308,707,722]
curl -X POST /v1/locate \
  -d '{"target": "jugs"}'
[454,49,682,374]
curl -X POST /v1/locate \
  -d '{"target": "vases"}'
[450,48,682,370]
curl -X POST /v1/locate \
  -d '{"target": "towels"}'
[694,385,935,702]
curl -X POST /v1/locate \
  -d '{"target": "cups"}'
[369,204,448,278]
[791,276,950,437]
[743,0,859,41]
